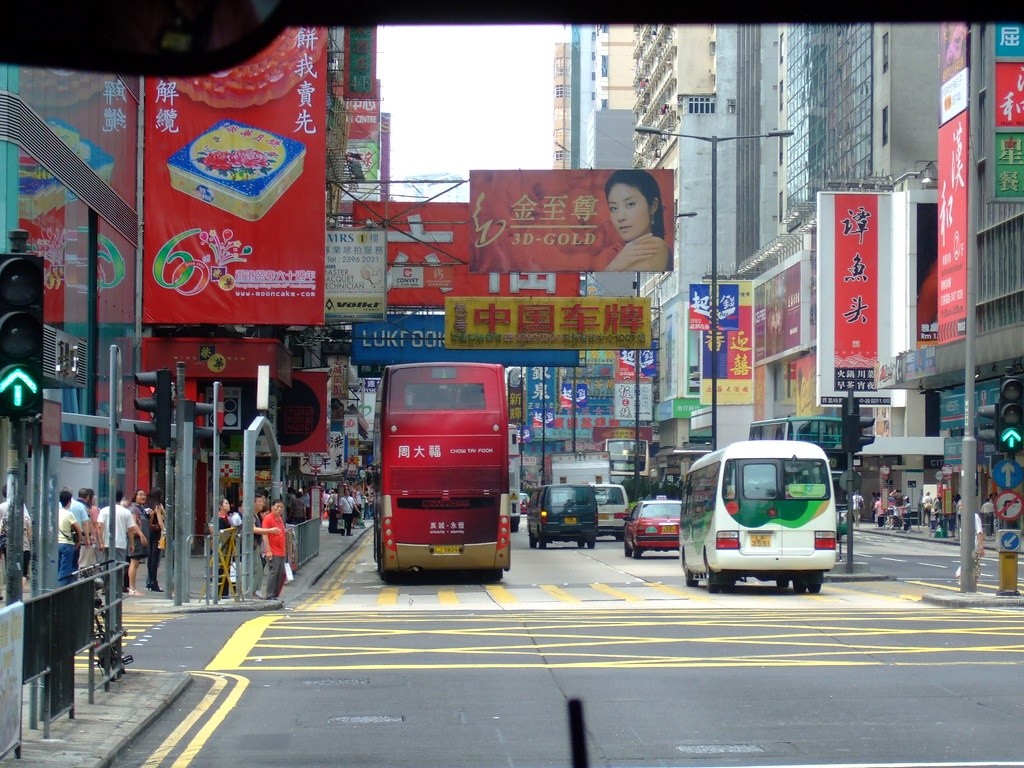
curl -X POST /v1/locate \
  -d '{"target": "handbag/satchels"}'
[337,519,345,529]
[859,500,864,509]
[157,532,166,549]
[283,562,293,584]
[353,509,359,517]
[72,531,77,542]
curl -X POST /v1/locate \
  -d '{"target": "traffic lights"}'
[977,403,998,449]
[994,375,1024,452]
[134,368,171,450]
[848,413,875,454]
[0,253,43,418]
[193,401,213,448]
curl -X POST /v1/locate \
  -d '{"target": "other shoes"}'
[347,534,352,536]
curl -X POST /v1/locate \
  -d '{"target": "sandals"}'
[129,589,143,595]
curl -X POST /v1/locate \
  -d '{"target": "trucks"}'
[544,451,611,484]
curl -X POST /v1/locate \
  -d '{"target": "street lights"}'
[635,125,795,452]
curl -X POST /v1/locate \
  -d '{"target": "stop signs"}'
[216,383,224,434]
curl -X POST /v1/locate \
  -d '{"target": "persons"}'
[852,489,1001,536]
[339,490,361,536]
[262,499,286,601]
[0,479,376,605]
[604,170,670,271]
[127,489,149,596]
[289,493,307,524]
[57,490,82,586]
[957,499,985,591]
[852,490,865,528]
[147,487,167,592]
[97,487,134,595]
[209,498,231,598]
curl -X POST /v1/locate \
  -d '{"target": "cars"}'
[623,495,686,559]
[520,500,530,514]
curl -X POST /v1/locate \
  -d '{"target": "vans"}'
[589,483,629,541]
[528,484,598,549]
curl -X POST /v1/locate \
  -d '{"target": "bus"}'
[508,424,520,532]
[373,362,510,581]
[679,440,837,593]
[599,438,650,485]
[748,416,848,541]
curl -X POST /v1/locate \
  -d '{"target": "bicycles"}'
[58,559,134,682]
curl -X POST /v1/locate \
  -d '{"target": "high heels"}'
[147,582,162,591]
